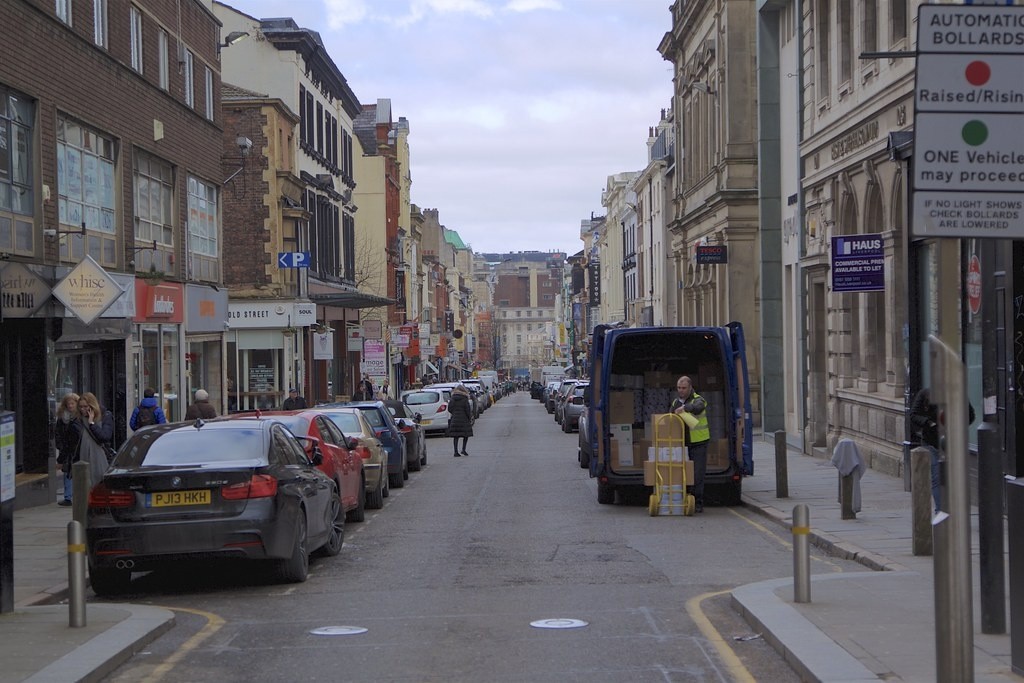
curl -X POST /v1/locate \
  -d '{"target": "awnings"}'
[448,363,461,371]
[459,366,473,373]
[426,361,439,374]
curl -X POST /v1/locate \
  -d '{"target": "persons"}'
[910,386,975,514]
[55,392,81,507]
[70,392,114,488]
[353,384,372,401]
[130,387,166,432]
[283,388,308,410]
[668,375,710,513]
[403,373,458,390]
[504,377,529,397]
[448,384,473,457]
[377,379,395,400]
[361,373,379,401]
[184,389,217,420]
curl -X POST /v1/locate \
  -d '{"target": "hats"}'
[289,388,297,392]
[195,389,208,402]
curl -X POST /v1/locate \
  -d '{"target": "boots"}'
[454,448,460,457]
[460,447,468,455]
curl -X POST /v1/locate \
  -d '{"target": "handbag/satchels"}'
[101,445,116,459]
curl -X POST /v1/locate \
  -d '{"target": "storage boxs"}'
[606,360,730,515]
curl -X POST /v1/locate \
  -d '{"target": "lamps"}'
[216,31,250,54]
[691,82,717,97]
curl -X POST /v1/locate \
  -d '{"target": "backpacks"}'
[136,404,159,430]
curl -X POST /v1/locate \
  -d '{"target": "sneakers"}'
[57,500,72,508]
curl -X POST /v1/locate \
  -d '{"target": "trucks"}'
[577,321,754,508]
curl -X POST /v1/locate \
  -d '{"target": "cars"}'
[213,409,371,524]
[378,399,428,472]
[399,368,520,439]
[81,414,347,599]
[312,398,408,489]
[529,319,590,434]
[296,406,391,509]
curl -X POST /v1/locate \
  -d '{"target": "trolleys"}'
[647,411,697,518]
